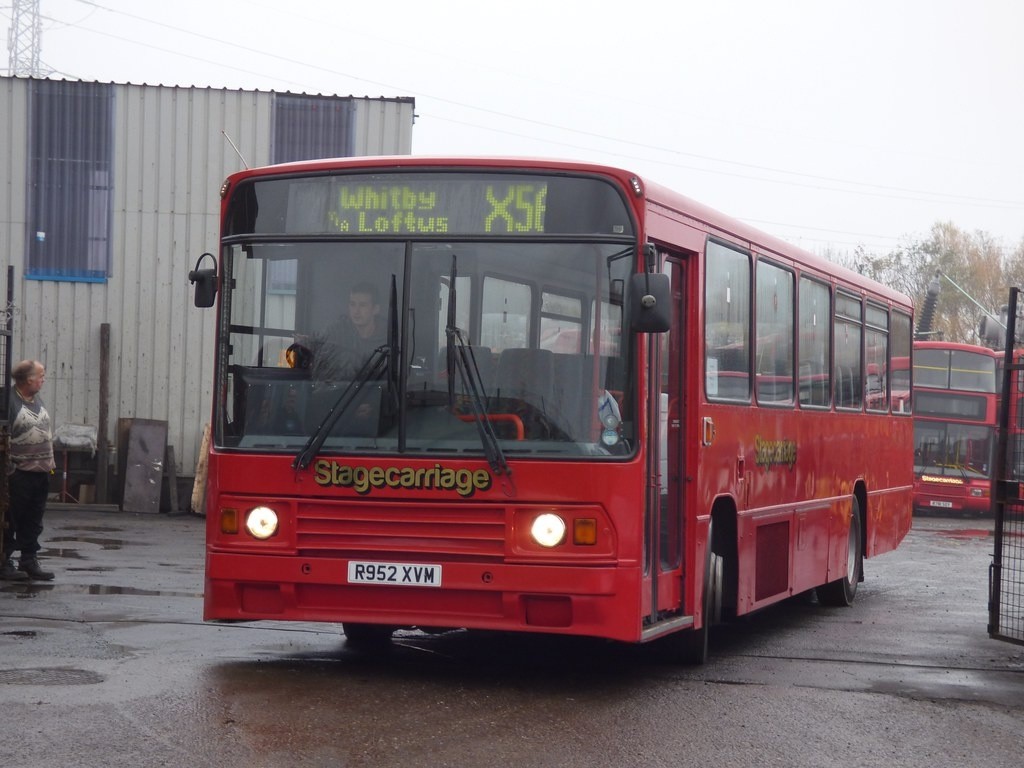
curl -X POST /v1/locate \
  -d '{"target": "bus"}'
[711,368,830,410]
[186,154,914,670]
[864,356,910,414]
[911,339,1024,519]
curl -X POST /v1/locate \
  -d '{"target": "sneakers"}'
[0,557,28,580]
[18,557,55,580]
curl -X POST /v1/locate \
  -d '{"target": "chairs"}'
[954,440,976,459]
[435,344,867,419]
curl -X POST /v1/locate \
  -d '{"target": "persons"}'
[0,358,56,582]
[312,289,393,382]
